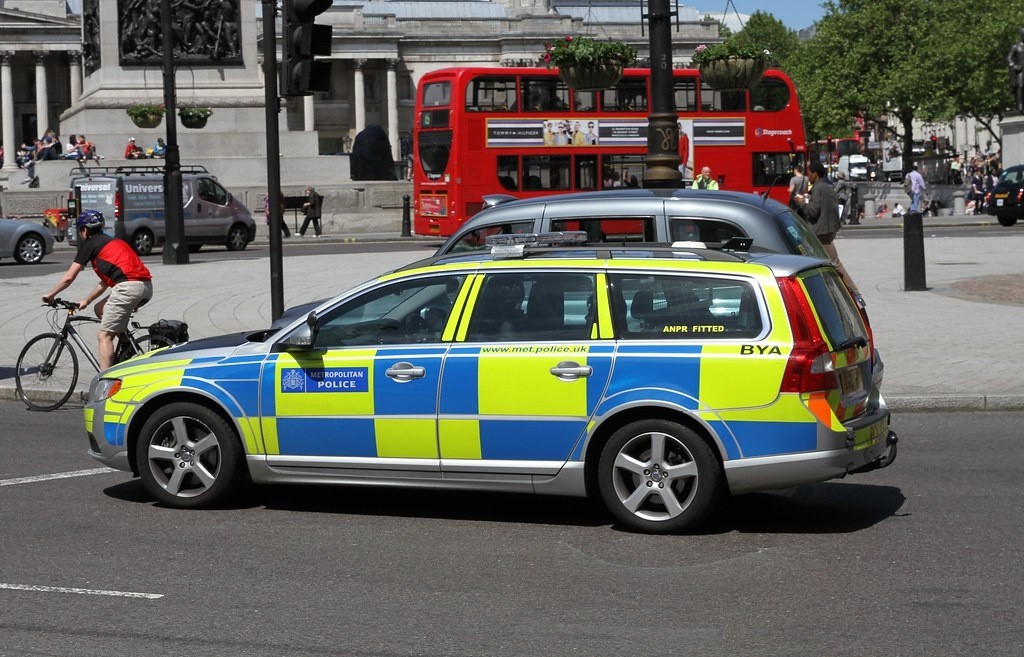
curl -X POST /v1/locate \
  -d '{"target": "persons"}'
[125,137,166,158]
[66,134,86,162]
[571,121,598,144]
[788,164,808,210]
[986,168,999,191]
[263,190,291,238]
[121,0,240,60]
[691,166,719,190]
[905,166,928,212]
[78,136,105,159]
[876,203,889,218]
[16,129,62,168]
[82,0,100,67]
[563,120,573,144]
[972,171,985,214]
[834,171,848,224]
[42,210,153,400]
[794,160,866,306]
[545,122,568,144]
[294,186,322,238]
[604,170,638,186]
[892,203,907,217]
[950,151,1000,186]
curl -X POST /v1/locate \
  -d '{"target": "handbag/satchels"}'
[840,193,848,200]
[148,319,189,346]
[790,191,802,209]
[968,189,975,199]
[301,205,309,212]
[904,172,911,193]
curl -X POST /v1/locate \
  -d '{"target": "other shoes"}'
[313,234,320,238]
[25,177,30,182]
[294,232,302,237]
[93,155,97,159]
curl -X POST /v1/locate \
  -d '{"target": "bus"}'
[811,139,864,164]
[414,58,810,245]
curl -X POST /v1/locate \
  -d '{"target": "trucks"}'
[881,139,926,182]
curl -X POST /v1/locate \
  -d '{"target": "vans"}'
[67,164,256,256]
[837,156,872,180]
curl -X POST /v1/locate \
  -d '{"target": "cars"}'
[270,189,868,344]
[0,217,55,264]
[985,165,1024,226]
[82,231,900,536]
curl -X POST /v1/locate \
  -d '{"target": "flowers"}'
[691,39,776,70]
[541,33,638,66]
[127,102,213,118]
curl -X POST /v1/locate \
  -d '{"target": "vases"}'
[699,58,767,90]
[131,114,162,128]
[557,59,623,91]
[180,116,208,128]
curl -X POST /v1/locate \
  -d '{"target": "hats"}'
[838,171,845,178]
[46,137,52,142]
[129,137,135,142]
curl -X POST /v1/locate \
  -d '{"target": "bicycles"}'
[15,298,178,413]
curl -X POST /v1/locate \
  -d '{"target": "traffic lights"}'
[282,0,333,97]
[827,135,833,152]
[931,136,937,149]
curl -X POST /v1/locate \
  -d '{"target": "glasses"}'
[588,126,593,128]
[558,126,563,128]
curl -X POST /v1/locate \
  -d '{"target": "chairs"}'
[493,105,642,111]
[498,176,543,190]
[198,183,216,203]
[474,276,565,334]
[631,286,714,333]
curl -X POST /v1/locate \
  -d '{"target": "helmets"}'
[73,210,105,228]
